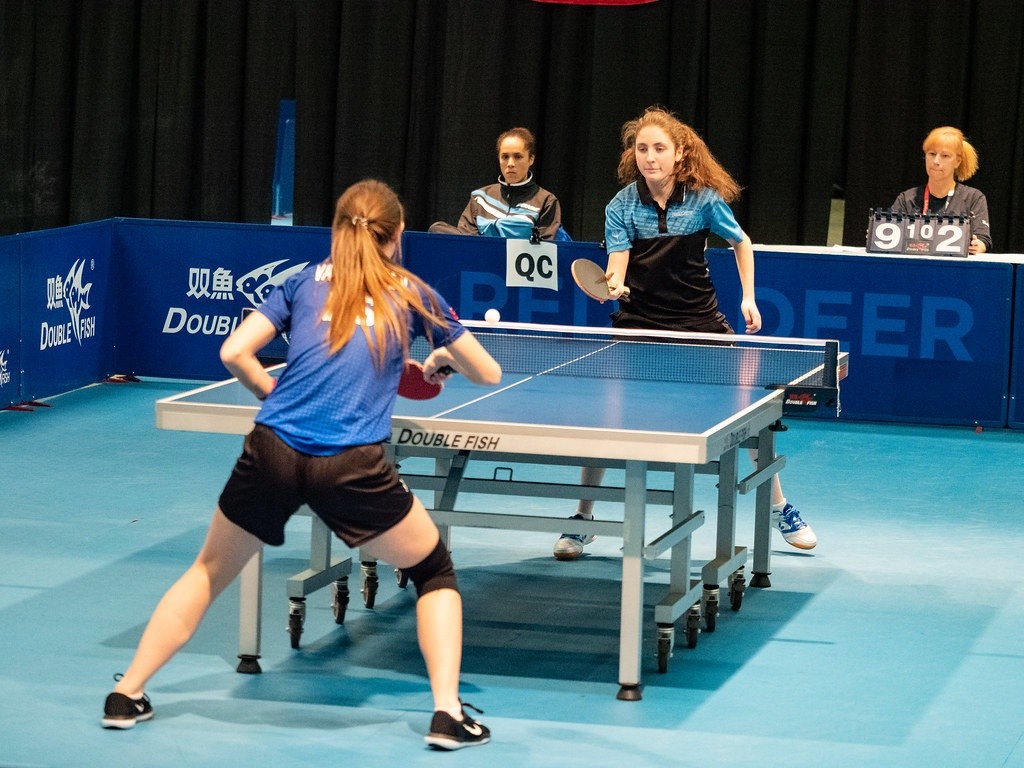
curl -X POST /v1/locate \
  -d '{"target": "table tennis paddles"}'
[571,258,630,302]
[397,360,449,400]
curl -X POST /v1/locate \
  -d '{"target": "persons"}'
[102,177,502,752]
[865,126,995,254]
[553,110,820,560]
[428,127,562,240]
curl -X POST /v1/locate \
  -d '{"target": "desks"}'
[155,331,849,700]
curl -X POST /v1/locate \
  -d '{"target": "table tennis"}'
[484,308,500,322]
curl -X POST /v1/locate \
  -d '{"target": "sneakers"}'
[772,503,817,550]
[424,697,491,751]
[553,514,598,558]
[101,673,154,730]
